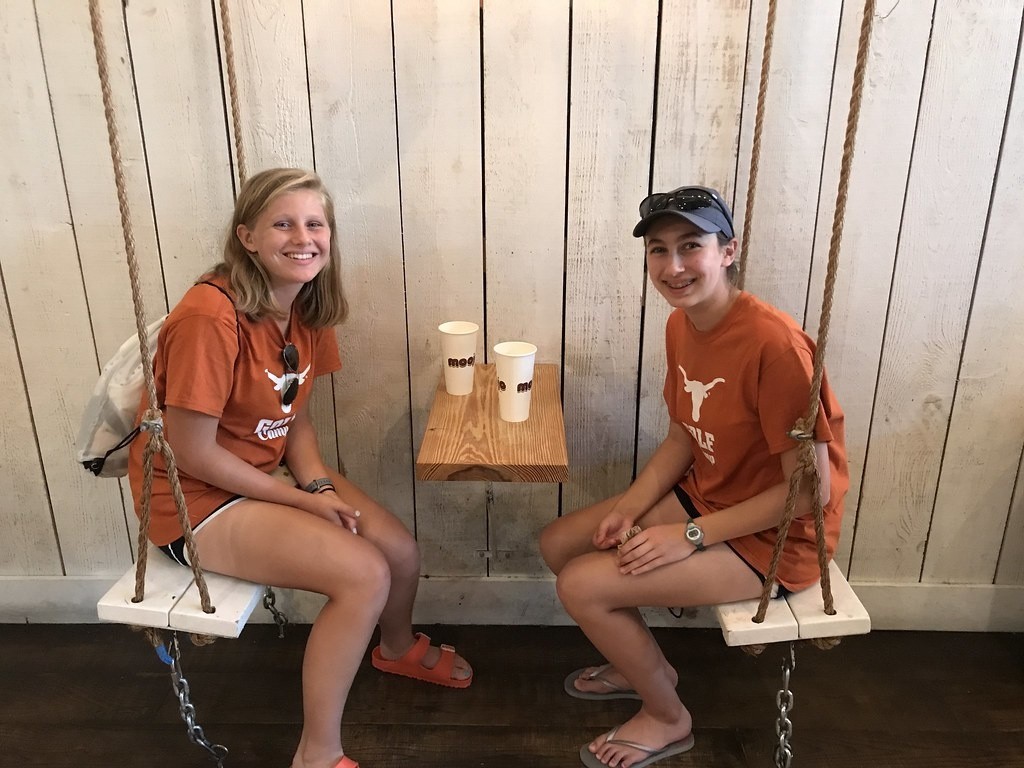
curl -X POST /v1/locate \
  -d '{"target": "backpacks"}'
[75,281,240,477]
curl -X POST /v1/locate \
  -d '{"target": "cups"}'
[494,342,537,422]
[438,321,479,395]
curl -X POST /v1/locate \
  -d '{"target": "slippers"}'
[564,664,643,700]
[371,632,473,688]
[579,724,694,768]
[334,755,360,768]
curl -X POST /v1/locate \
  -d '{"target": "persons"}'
[536,185,854,768]
[129,165,474,768]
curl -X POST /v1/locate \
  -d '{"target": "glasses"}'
[282,344,301,406]
[639,188,735,237]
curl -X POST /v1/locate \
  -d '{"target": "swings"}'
[710,0,872,643]
[80,0,304,640]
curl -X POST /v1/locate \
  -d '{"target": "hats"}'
[632,186,734,238]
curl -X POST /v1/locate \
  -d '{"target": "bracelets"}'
[304,478,335,493]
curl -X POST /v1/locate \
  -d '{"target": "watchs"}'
[685,518,706,551]
